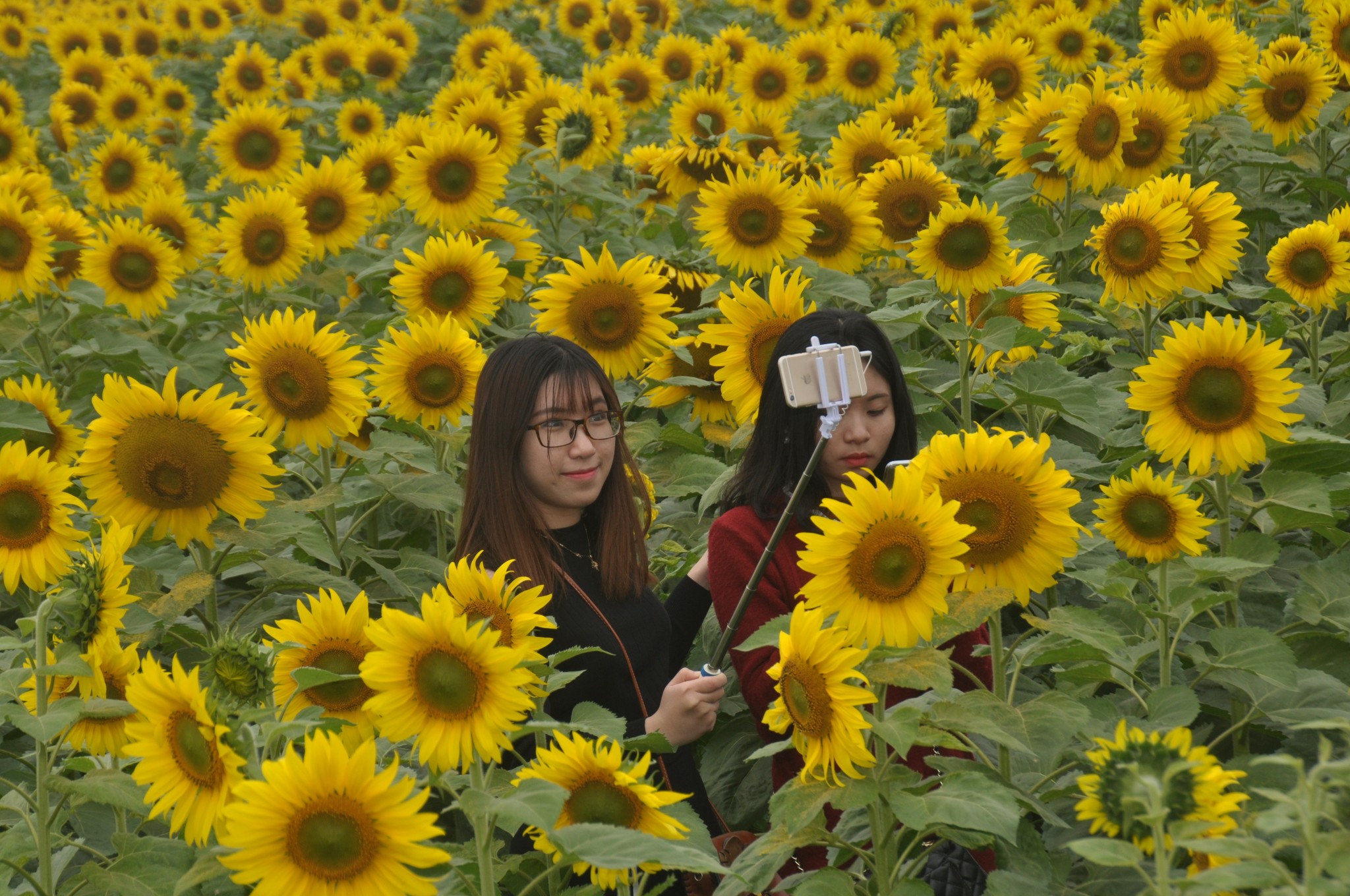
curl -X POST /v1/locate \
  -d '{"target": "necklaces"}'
[531,518,600,574]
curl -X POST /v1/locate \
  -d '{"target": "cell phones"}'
[777,344,868,409]
[884,460,908,489]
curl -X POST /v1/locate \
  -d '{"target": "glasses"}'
[527,410,624,448]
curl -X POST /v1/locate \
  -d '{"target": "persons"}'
[707,305,999,877]
[454,329,727,896]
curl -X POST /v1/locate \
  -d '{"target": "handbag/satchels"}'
[682,830,791,896]
[853,835,989,896]
